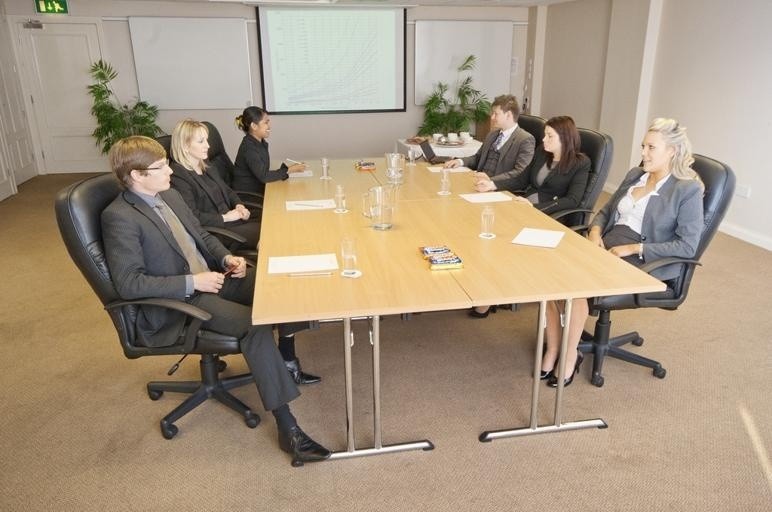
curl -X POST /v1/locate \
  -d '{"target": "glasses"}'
[135,159,169,170]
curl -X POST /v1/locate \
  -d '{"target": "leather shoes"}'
[282,358,321,385]
[278,426,330,461]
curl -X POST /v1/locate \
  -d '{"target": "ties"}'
[154,197,205,273]
[495,133,503,148]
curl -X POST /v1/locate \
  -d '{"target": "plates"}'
[436,143,464,147]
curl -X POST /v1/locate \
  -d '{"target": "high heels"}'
[469,306,496,318]
[546,348,584,387]
[533,354,558,380]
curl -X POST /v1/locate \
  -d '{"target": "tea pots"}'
[362,183,400,229]
[384,152,405,183]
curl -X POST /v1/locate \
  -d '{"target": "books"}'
[419,244,464,271]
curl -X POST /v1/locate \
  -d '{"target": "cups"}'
[448,133,457,140]
[481,205,493,236]
[321,158,330,177]
[341,238,355,276]
[460,132,469,139]
[334,184,345,211]
[433,134,443,142]
[463,137,472,142]
[408,148,415,165]
[440,169,450,192]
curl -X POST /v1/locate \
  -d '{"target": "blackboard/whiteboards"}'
[414,19,514,106]
[127,16,252,110]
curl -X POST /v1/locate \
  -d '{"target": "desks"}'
[398,135,482,165]
[259,155,474,463]
[363,157,666,441]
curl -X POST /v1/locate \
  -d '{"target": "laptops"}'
[418,139,452,165]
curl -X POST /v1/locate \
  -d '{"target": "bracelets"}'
[638,242,644,261]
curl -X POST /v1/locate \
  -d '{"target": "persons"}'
[100,136,331,462]
[443,95,536,193]
[531,117,704,387]
[169,118,261,250]
[230,106,305,216]
[467,115,591,318]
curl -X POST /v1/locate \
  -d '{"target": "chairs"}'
[54,173,277,440]
[200,121,264,199]
[517,114,549,147]
[514,126,612,225]
[155,133,261,258]
[556,145,736,387]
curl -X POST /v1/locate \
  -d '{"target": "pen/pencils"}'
[223,261,241,276]
[287,159,308,167]
[289,273,330,275]
[296,204,323,207]
